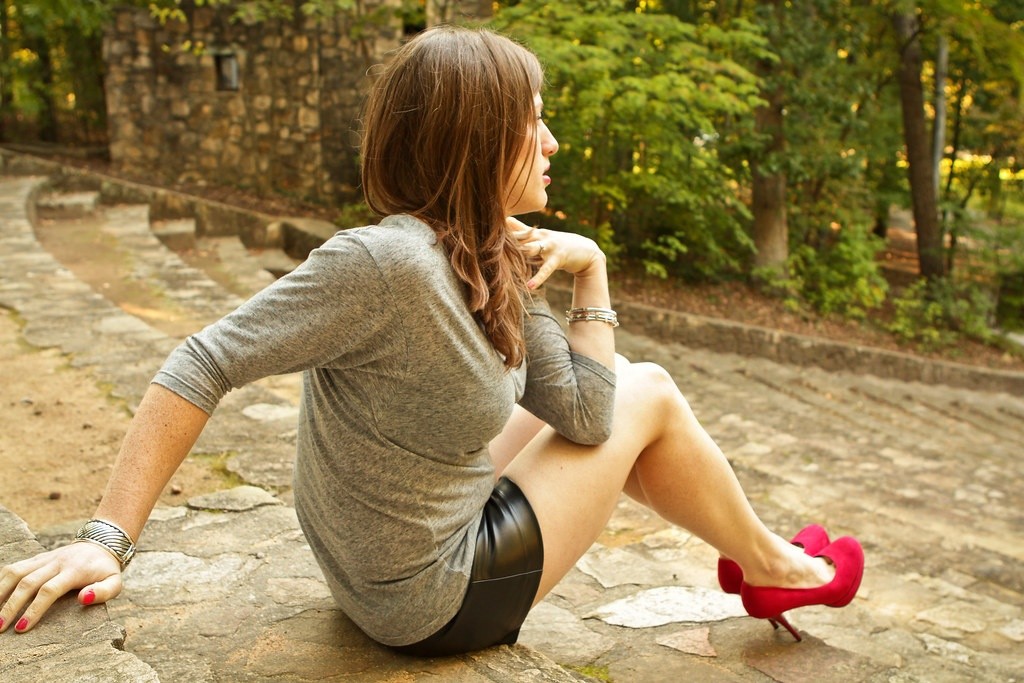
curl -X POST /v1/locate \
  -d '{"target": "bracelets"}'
[566,309,620,328]
[73,519,138,571]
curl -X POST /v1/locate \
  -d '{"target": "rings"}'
[537,239,545,255]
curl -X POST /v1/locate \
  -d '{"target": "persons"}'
[0,24,865,645]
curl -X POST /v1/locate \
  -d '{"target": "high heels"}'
[718,525,831,630]
[740,535,865,641]
